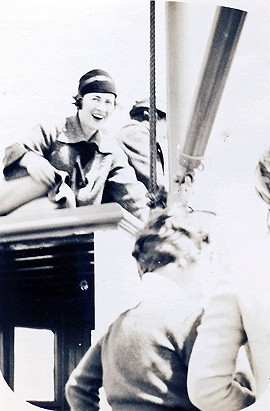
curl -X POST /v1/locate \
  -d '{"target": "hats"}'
[129,103,166,118]
[79,69,117,98]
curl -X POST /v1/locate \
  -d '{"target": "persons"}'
[186,142,270,411]
[66,207,257,411]
[0,68,166,228]
